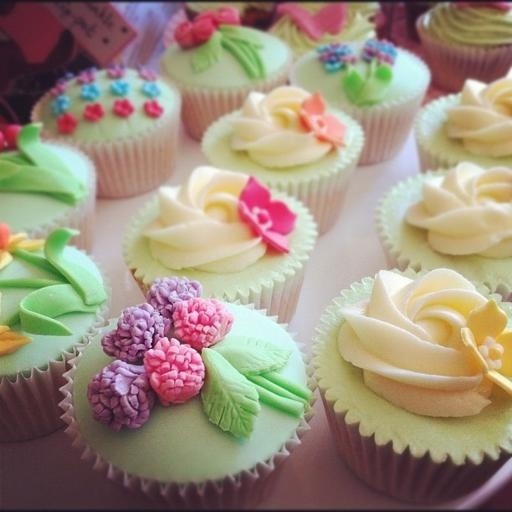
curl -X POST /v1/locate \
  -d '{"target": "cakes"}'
[376,160,512,309]
[30,65,180,200]
[198,85,364,237]
[314,267,512,504]
[2,227,107,448]
[122,165,317,329]
[0,118,94,255]
[62,276,314,509]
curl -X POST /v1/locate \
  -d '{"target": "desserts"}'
[160,0,512,173]
